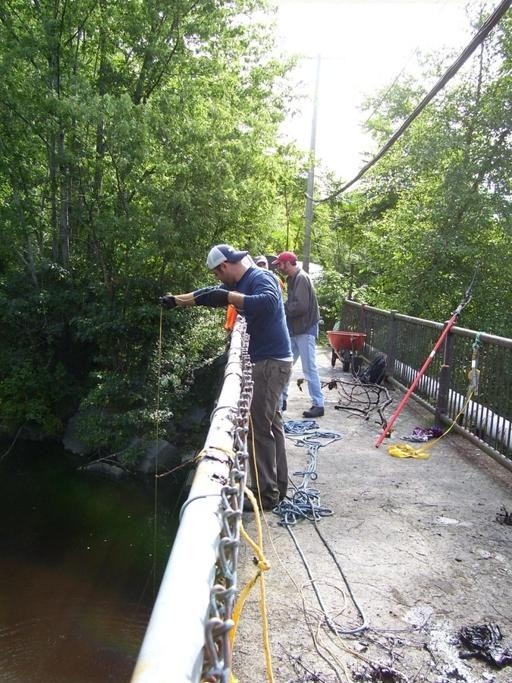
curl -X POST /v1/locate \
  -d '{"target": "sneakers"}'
[242,497,272,512]
[303,406,324,417]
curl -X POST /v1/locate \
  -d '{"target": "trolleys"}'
[327,331,367,371]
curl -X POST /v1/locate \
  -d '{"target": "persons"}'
[271,251,325,418]
[253,255,285,291]
[159,244,294,511]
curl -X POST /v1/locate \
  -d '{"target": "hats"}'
[253,255,268,265]
[207,244,249,273]
[271,252,298,265]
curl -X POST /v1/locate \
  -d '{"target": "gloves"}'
[158,296,178,311]
[202,289,230,308]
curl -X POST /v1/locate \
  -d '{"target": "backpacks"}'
[360,355,387,385]
